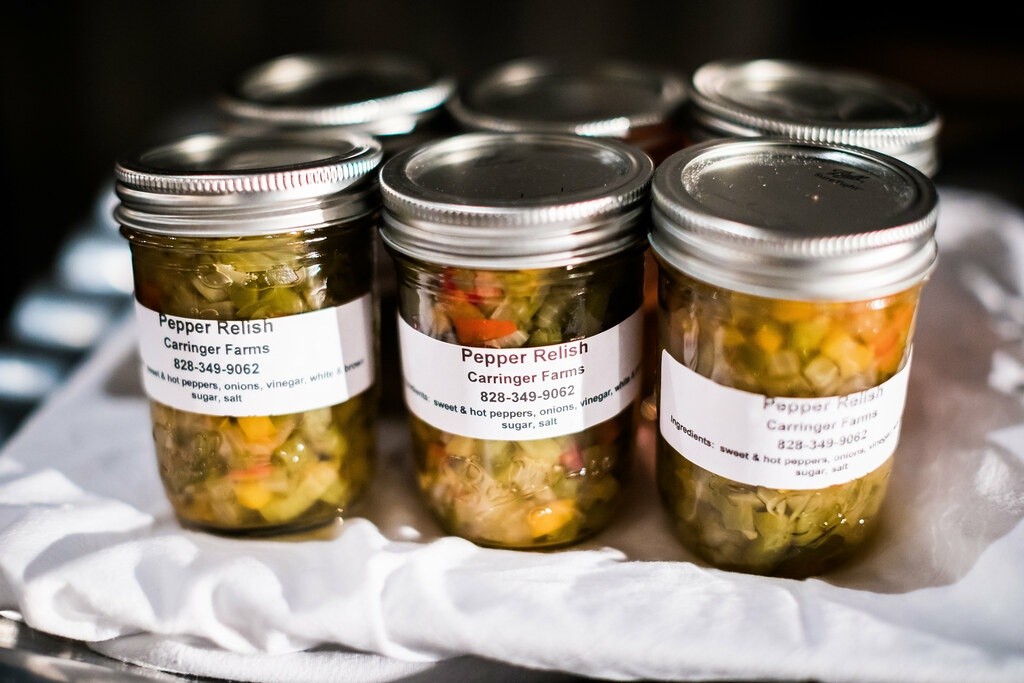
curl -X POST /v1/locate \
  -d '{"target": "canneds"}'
[114,51,945,580]
